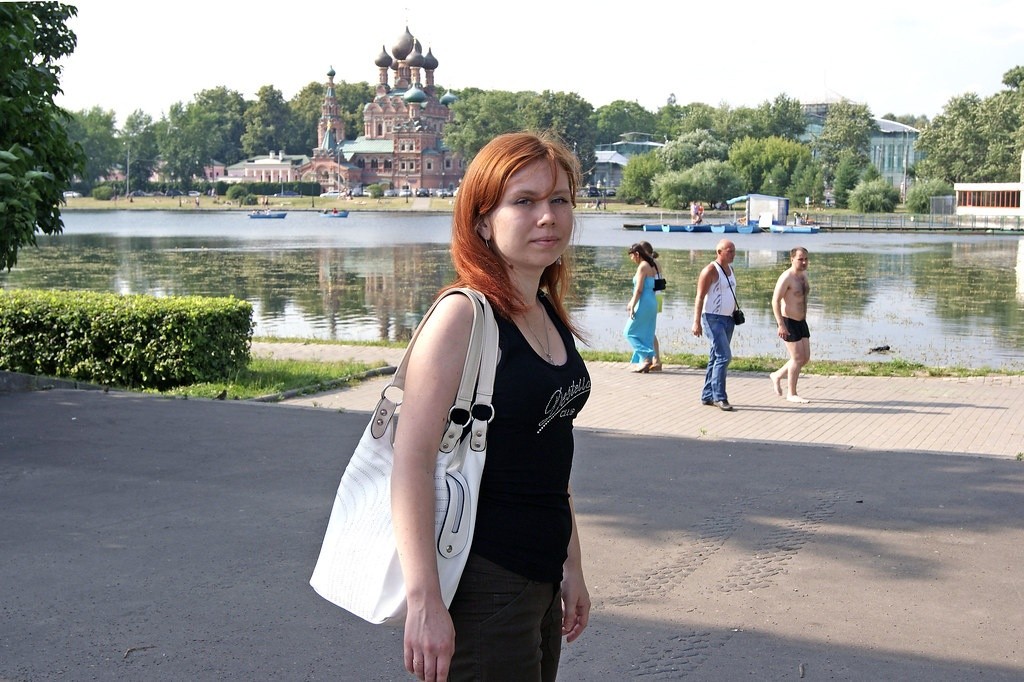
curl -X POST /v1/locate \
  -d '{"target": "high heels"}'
[631,363,650,374]
[650,363,663,372]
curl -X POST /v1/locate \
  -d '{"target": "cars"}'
[383,189,397,197]
[436,189,447,197]
[189,191,200,197]
[351,187,363,196]
[416,188,430,197]
[399,189,412,197]
[63,190,81,197]
[606,188,617,196]
[130,191,147,197]
[274,191,299,197]
[320,190,342,198]
[166,189,183,195]
[449,188,459,197]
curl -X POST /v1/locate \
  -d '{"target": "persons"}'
[390,136,591,682]
[595,198,601,211]
[261,196,268,208]
[770,247,811,404]
[342,187,353,200]
[623,241,662,373]
[691,201,704,222]
[252,209,260,214]
[794,211,798,224]
[333,208,337,213]
[692,239,736,410]
[324,208,328,214]
[266,208,271,215]
[195,196,199,207]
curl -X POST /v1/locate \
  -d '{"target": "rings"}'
[413,660,423,665]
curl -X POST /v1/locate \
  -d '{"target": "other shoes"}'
[701,400,715,405]
[714,399,732,412]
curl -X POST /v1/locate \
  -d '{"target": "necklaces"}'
[522,296,555,365]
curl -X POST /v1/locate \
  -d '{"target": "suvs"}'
[588,187,601,197]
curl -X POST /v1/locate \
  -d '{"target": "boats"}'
[770,223,820,234]
[248,212,287,218]
[319,211,349,218]
[623,222,763,234]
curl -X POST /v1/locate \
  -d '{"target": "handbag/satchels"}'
[654,278,667,291]
[734,310,745,326]
[310,287,499,630]
